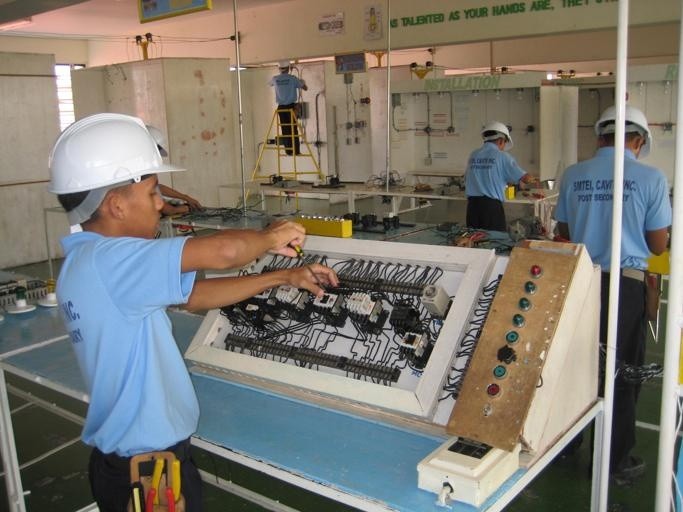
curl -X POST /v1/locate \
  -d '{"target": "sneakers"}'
[609,453,646,480]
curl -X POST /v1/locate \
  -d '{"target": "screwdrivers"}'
[266,223,328,293]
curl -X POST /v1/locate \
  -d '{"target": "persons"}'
[464,120,530,233]
[46,114,338,512]
[551,105,675,477]
[268,60,308,155]
[147,125,202,217]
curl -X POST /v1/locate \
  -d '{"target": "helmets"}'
[277,60,289,68]
[44,111,188,195]
[144,124,169,157]
[594,105,652,158]
[480,121,513,150]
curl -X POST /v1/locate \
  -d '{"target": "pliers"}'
[145,458,181,512]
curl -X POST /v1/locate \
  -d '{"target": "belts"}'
[621,266,647,285]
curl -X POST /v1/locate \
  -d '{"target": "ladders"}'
[245,108,326,209]
